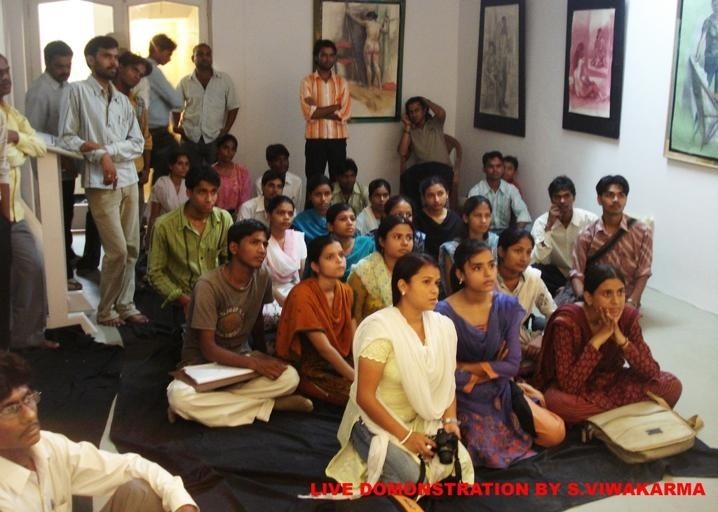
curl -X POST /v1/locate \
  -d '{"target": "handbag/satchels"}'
[584,392,704,464]
[553,273,579,307]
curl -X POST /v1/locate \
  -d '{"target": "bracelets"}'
[402,128,410,134]
[616,336,628,347]
[443,418,461,426]
[400,430,412,444]
[627,298,639,308]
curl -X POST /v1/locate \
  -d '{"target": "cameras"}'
[426,428,458,465]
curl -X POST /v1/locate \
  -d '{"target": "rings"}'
[107,174,111,178]
[115,175,119,180]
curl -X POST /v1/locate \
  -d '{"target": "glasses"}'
[0,390,43,419]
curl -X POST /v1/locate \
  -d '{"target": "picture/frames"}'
[663,1,718,170]
[313,0,406,123]
[562,0,626,138]
[474,0,525,138]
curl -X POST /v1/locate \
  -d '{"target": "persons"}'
[364,194,427,253]
[262,195,308,329]
[573,41,600,98]
[348,214,415,334]
[349,251,475,494]
[590,29,607,69]
[0,350,200,512]
[569,175,653,313]
[173,43,240,166]
[694,0,718,100]
[287,175,335,244]
[469,151,533,231]
[210,134,251,215]
[166,219,314,428]
[0,52,60,351]
[356,178,395,237]
[0,108,7,349]
[143,34,184,152]
[415,176,466,255]
[235,170,286,236]
[398,95,454,198]
[500,156,522,197]
[330,157,370,217]
[147,165,234,320]
[301,39,350,208]
[433,238,566,470]
[112,52,154,222]
[438,196,500,300]
[345,9,389,92]
[530,175,599,300]
[275,231,355,405]
[146,152,190,255]
[327,203,376,279]
[484,40,505,111]
[25,40,84,291]
[494,229,560,376]
[539,264,682,445]
[497,15,508,58]
[255,144,302,209]
[58,35,148,327]
